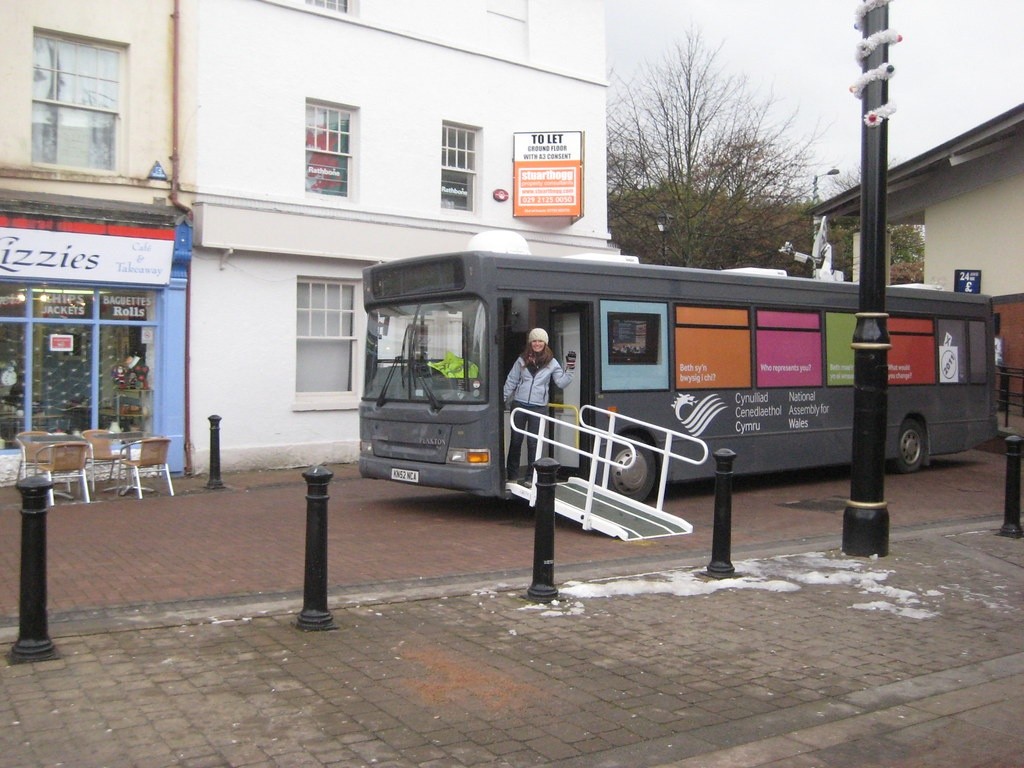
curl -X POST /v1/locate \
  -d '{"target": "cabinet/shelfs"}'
[98,387,154,434]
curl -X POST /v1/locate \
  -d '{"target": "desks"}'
[18,434,92,500]
[94,431,183,496]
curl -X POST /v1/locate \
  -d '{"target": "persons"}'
[503,328,576,488]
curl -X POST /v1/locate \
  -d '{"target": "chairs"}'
[15,431,65,492]
[35,441,91,509]
[115,437,174,499]
[81,430,130,500]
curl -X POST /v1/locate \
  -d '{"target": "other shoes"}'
[523,479,532,488]
[505,479,517,483]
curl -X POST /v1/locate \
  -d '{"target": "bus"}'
[350,247,999,543]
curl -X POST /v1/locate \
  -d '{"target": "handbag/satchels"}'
[504,391,515,410]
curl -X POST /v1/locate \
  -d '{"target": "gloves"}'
[565,350,576,369]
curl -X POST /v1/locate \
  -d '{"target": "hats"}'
[528,328,549,345]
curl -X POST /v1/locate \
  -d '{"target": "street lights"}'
[813,167,842,205]
